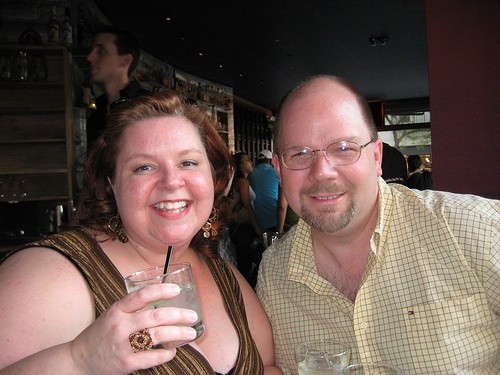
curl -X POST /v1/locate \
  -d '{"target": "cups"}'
[294,339,351,375]
[123,262,205,351]
[342,362,403,375]
[262,232,286,248]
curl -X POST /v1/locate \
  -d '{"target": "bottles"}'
[60,7,73,44]
[48,5,60,42]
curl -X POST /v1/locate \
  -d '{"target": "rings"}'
[129,327,153,354]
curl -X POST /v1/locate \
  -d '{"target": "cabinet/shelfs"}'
[0,44,273,269]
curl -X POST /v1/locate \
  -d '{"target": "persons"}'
[254,74,500,375]
[0,88,285,375]
[83,23,432,290]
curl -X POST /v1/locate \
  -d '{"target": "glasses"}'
[278,140,373,170]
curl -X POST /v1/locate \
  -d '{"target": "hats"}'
[257,150,272,159]
